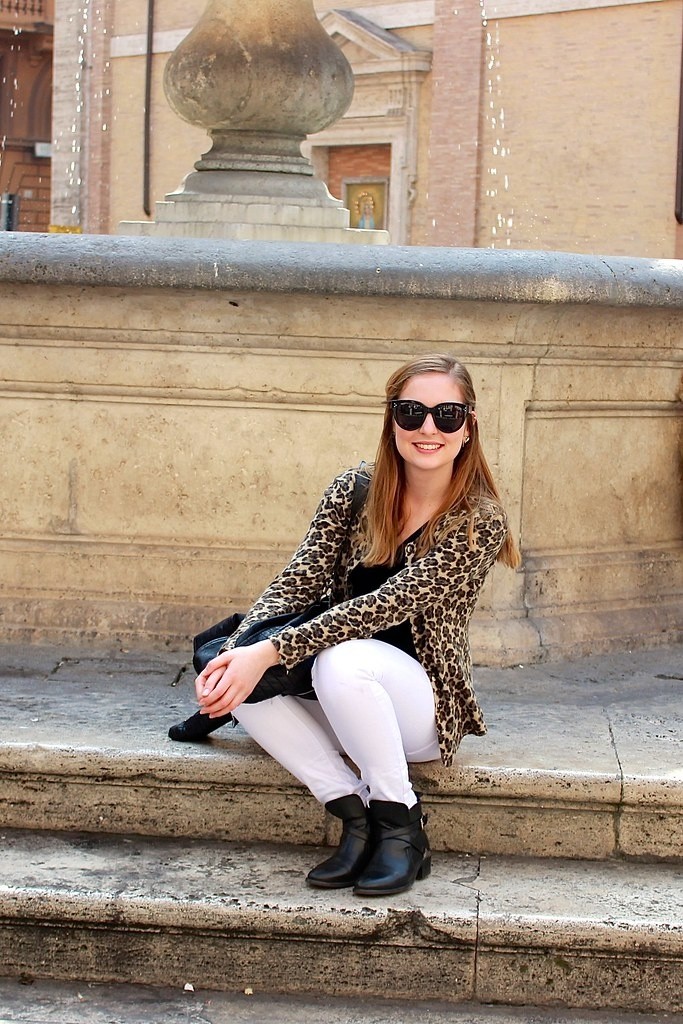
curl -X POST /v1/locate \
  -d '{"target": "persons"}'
[194,353,521,896]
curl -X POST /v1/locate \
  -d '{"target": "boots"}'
[353,791,432,895]
[304,793,374,889]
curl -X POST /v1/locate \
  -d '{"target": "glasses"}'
[391,400,474,433]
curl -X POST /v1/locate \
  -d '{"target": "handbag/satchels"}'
[168,599,335,743]
